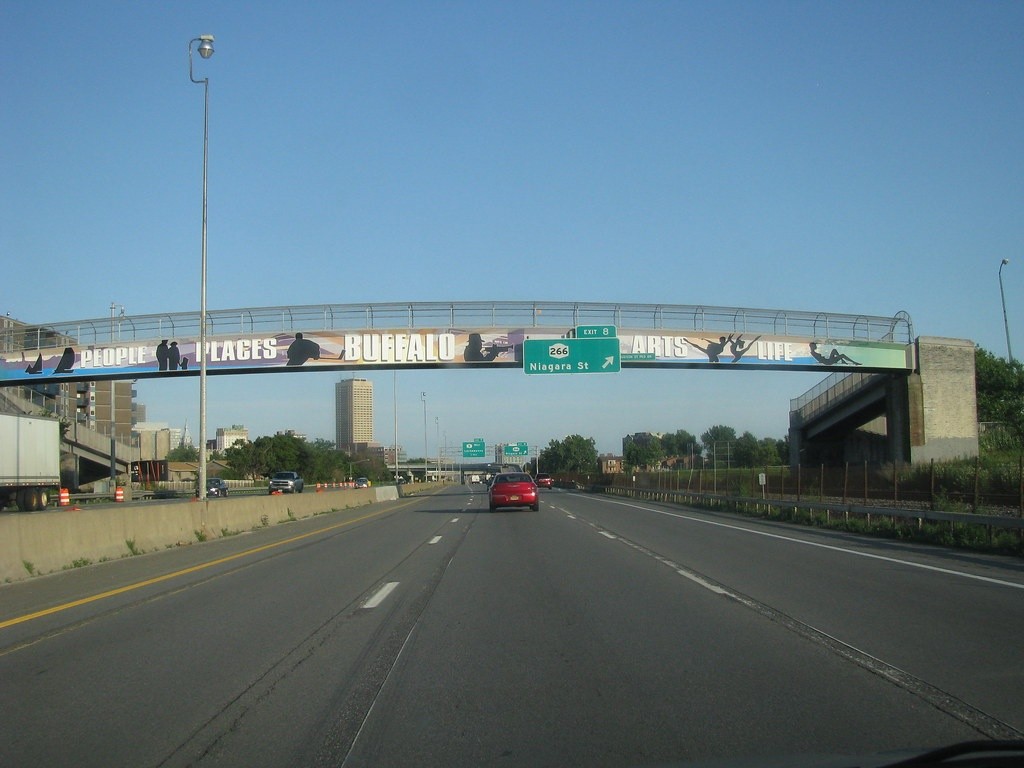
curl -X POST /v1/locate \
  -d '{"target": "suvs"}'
[268,470,304,495]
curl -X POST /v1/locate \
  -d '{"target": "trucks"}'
[0,413,61,512]
[472,474,481,484]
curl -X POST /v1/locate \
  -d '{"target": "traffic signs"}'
[504,441,529,457]
[521,337,622,376]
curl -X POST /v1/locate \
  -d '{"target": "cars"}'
[533,473,555,490]
[195,478,229,498]
[354,478,370,489]
[489,471,541,511]
[487,473,500,491]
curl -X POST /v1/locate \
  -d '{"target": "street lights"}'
[435,416,440,482]
[421,391,428,483]
[107,301,125,494]
[998,257,1017,366]
[186,35,217,510]
[349,459,371,483]
